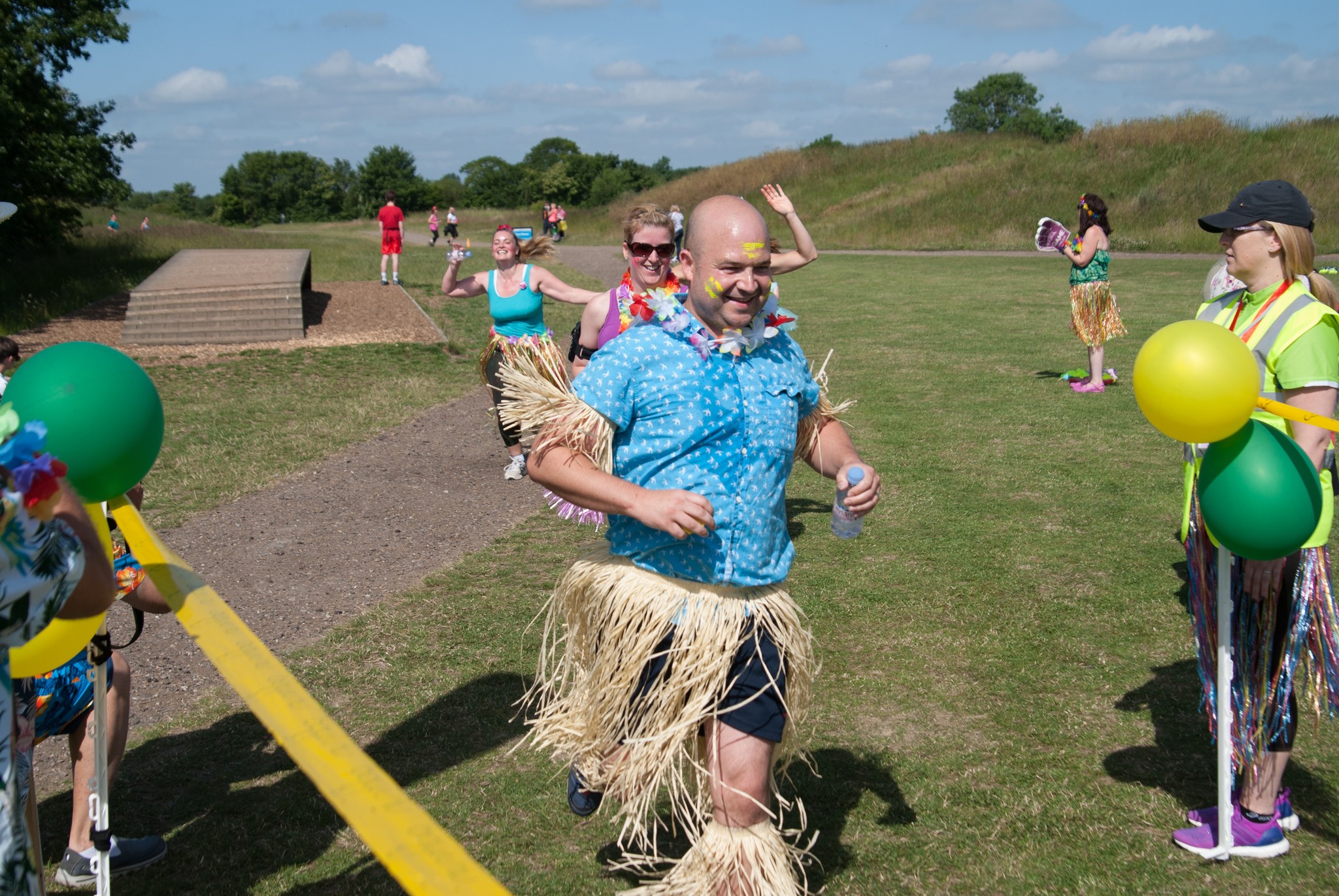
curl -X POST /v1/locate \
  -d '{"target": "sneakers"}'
[567,762,606,817]
[502,457,529,481]
[1181,781,1300,835]
[54,831,166,890]
[1171,802,1291,863]
[393,278,403,285]
[380,279,390,286]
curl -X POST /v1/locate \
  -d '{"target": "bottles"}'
[829,467,865,542]
[446,249,472,262]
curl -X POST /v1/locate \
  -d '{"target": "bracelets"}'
[1061,245,1068,255]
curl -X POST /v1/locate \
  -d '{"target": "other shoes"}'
[448,240,454,246]
[429,240,436,248]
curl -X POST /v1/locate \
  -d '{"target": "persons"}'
[0,336,21,398]
[523,191,886,896]
[1169,179,1338,860]
[669,183,818,289]
[1035,193,1128,393]
[667,203,684,263]
[443,206,458,246]
[440,224,609,480]
[542,201,566,242]
[0,400,112,895]
[567,203,692,380]
[35,534,172,891]
[427,206,442,246]
[377,191,404,286]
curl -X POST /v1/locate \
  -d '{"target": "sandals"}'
[1070,376,1094,388]
[1071,379,1107,393]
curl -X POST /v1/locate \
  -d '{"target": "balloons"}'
[1196,419,1322,561]
[1131,319,1258,444]
[2,340,164,503]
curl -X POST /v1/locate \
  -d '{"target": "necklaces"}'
[501,275,512,286]
[617,266,681,333]
[646,288,796,360]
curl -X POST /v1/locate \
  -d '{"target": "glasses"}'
[624,240,678,259]
[10,353,21,362]
[1224,223,1279,237]
[545,204,547,206]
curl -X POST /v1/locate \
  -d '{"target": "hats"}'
[1197,175,1316,241]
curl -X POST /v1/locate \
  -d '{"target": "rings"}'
[1263,570,1272,574]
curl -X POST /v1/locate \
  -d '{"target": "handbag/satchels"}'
[557,220,567,231]
[548,208,558,223]
